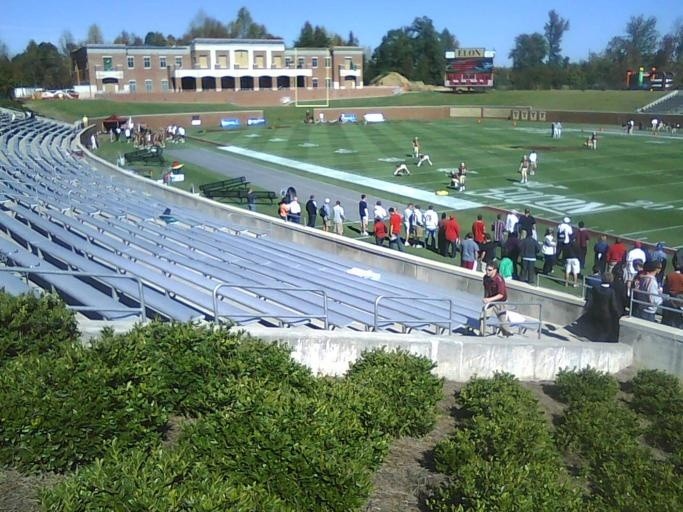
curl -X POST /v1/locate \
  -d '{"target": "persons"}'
[11,112,16,121]
[83,113,88,127]
[107,121,186,150]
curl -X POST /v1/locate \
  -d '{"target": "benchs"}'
[637,90,682,116]
[0,113,539,337]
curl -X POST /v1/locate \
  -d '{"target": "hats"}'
[525,209,529,213]
[563,218,570,223]
[377,201,381,206]
[635,242,642,247]
[633,259,642,266]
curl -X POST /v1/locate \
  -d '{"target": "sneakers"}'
[361,231,368,236]
[405,242,423,248]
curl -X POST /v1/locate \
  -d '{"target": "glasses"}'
[485,268,494,271]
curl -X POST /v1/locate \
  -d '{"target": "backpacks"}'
[320,206,326,217]
